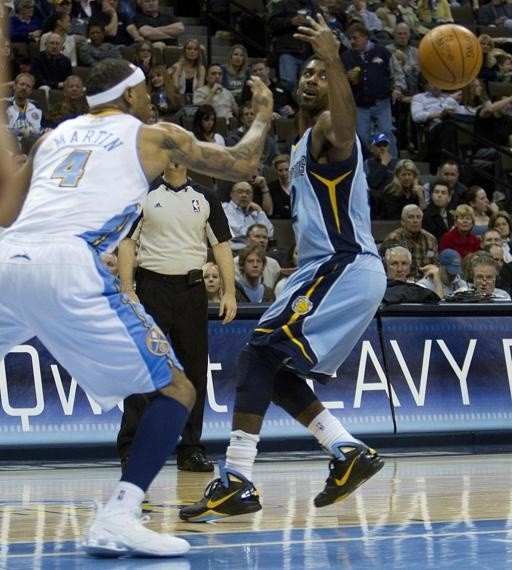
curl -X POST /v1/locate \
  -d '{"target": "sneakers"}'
[179,460,262,523]
[81,501,189,557]
[314,439,384,507]
[177,448,214,472]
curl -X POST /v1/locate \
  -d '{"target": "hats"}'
[439,249,462,275]
[369,133,390,145]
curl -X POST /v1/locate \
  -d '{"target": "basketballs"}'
[416,22,485,91]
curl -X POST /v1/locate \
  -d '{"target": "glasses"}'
[474,274,496,286]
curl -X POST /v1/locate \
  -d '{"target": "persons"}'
[0,58,275,559]
[178,10,390,525]
[116,149,242,478]
[1,2,512,309]
